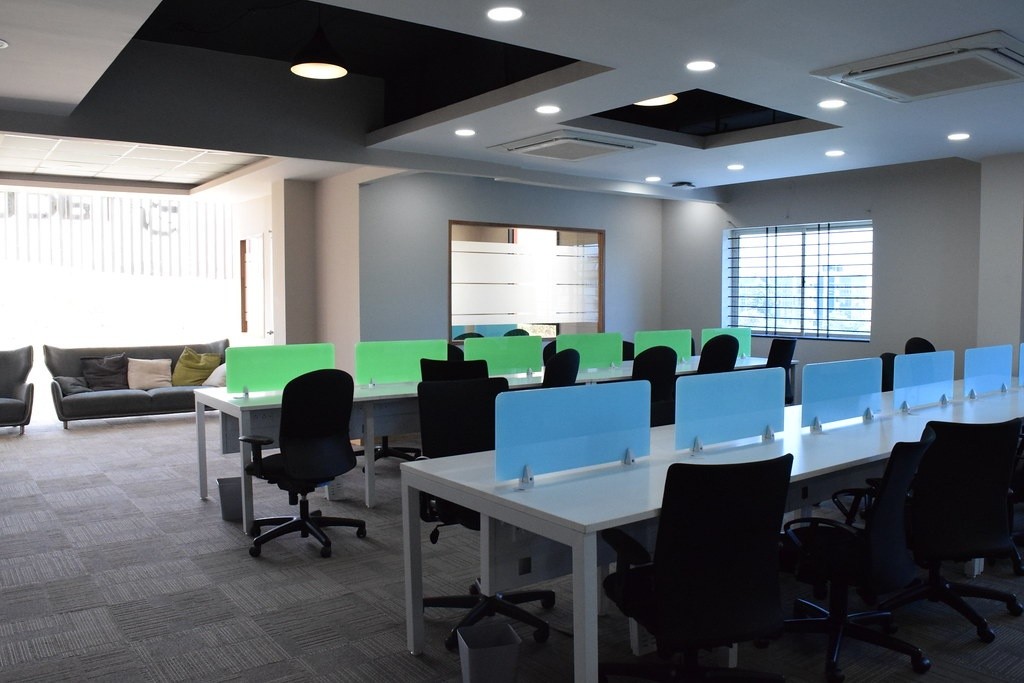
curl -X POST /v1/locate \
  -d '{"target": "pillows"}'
[172,346,223,386]
[202,363,226,387]
[127,357,172,389]
[80,352,130,391]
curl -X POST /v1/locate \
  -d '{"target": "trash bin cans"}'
[456,621,522,683]
[216,477,243,521]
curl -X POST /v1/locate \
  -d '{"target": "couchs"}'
[43,339,229,429]
[0,345,34,434]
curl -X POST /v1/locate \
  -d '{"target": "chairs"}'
[236,330,1024,683]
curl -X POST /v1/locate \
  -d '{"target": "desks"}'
[193,355,798,536]
[398,375,1024,683]
